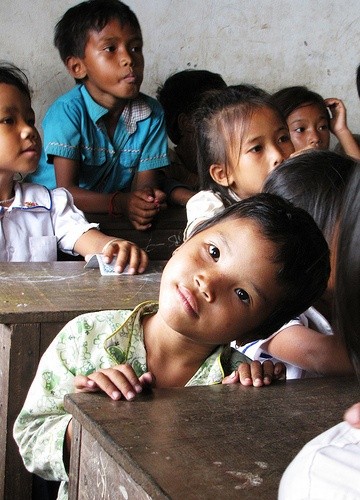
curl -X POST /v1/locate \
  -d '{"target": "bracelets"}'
[101,237,124,255]
[108,192,121,213]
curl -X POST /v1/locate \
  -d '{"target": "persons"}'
[0,60,149,277]
[232,148,360,381]
[155,70,229,205]
[181,84,295,241]
[269,84,360,168]
[11,194,332,500]
[275,401,360,500]
[22,1,171,231]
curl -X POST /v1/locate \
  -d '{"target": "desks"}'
[0,262,172,500]
[64,372,360,500]
